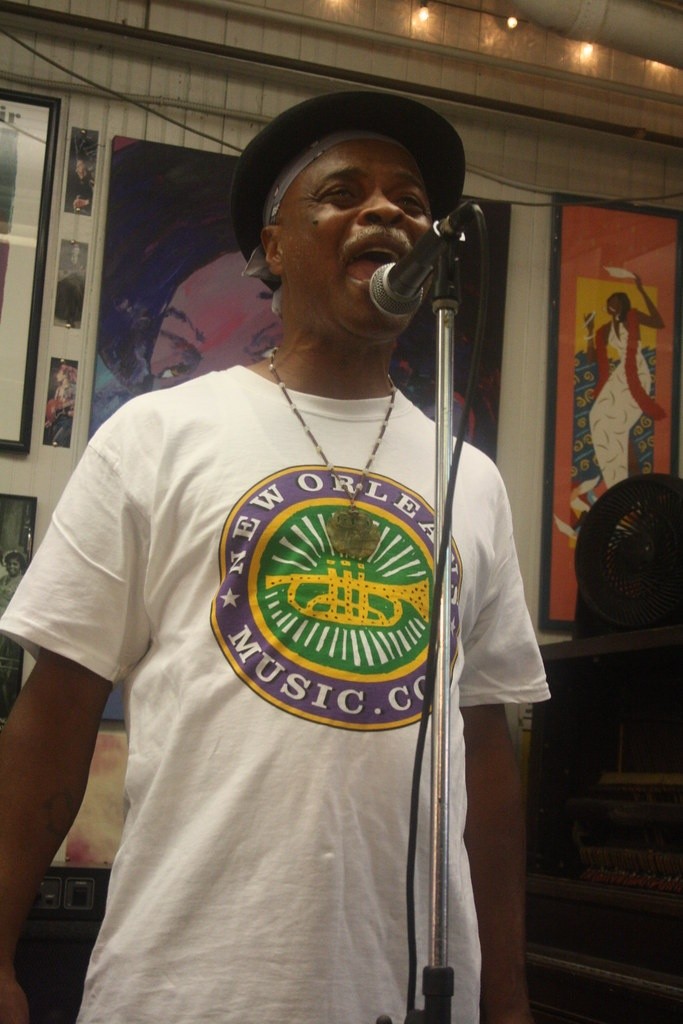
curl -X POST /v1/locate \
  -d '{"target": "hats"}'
[230,92,466,292]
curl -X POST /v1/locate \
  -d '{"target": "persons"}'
[0,89,550,1024]
[59,244,82,328]
[73,157,93,214]
[46,365,77,445]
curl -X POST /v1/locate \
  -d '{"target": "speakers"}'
[15,920,102,1024]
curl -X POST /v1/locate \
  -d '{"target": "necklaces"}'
[269,347,396,558]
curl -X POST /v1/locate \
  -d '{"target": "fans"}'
[575,476,683,628]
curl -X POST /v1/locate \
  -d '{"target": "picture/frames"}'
[0,85,64,451]
[0,493,38,712]
[539,190,683,629]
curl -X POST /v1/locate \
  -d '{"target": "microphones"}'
[368,201,476,319]
